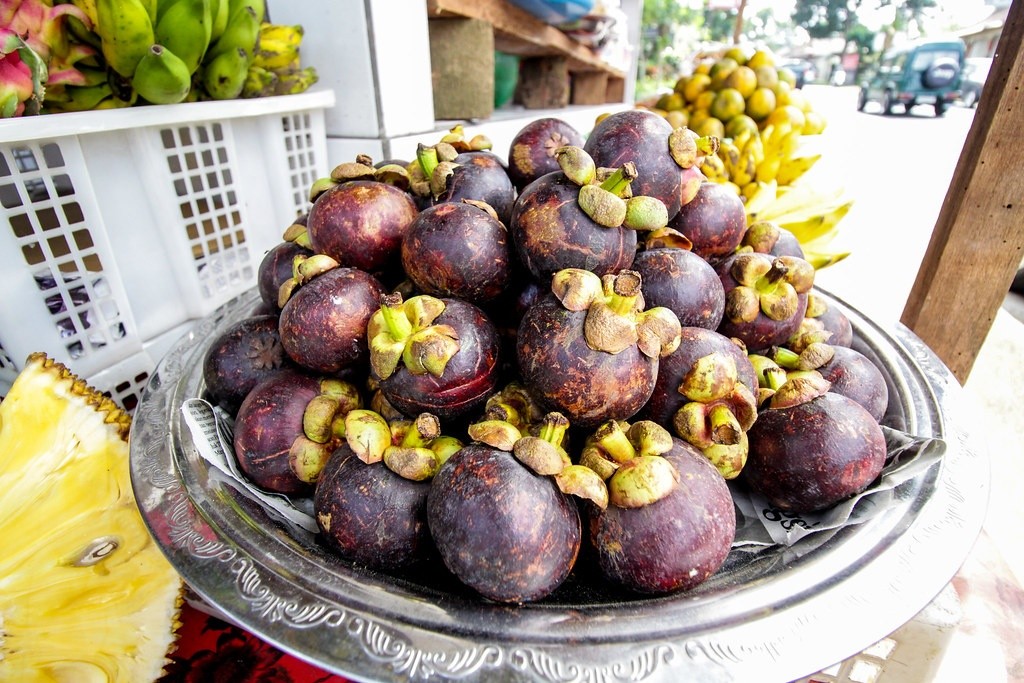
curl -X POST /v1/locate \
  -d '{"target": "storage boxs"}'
[0,85,342,420]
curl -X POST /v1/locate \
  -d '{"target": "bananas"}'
[688,121,854,270]
[40,0,320,114]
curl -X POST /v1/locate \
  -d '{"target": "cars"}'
[774,57,807,91]
[957,57,994,110]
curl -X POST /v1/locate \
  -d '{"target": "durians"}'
[0,352,189,683]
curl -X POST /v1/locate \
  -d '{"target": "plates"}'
[132,273,991,683]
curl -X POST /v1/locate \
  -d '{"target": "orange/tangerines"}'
[592,43,826,138]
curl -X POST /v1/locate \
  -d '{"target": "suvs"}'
[857,37,967,118]
